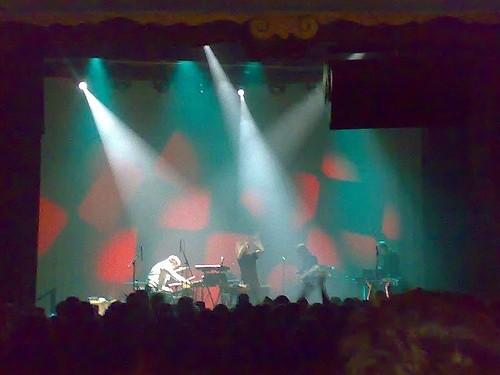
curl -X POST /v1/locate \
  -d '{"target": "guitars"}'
[294,265,320,286]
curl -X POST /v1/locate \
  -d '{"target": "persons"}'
[295,244,329,303]
[236,239,265,303]
[0,265,500,374]
[146,255,189,298]
[376,242,399,278]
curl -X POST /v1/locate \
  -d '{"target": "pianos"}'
[190,264,230,309]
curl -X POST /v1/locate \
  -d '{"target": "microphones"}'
[141,246,143,260]
[221,257,224,265]
[180,240,181,253]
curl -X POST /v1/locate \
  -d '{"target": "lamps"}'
[234,82,247,96]
[113,79,131,93]
[152,79,171,94]
[269,84,286,95]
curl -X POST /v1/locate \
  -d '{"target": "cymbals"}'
[119,281,150,285]
[175,266,189,272]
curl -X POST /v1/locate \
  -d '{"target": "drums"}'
[168,283,183,299]
